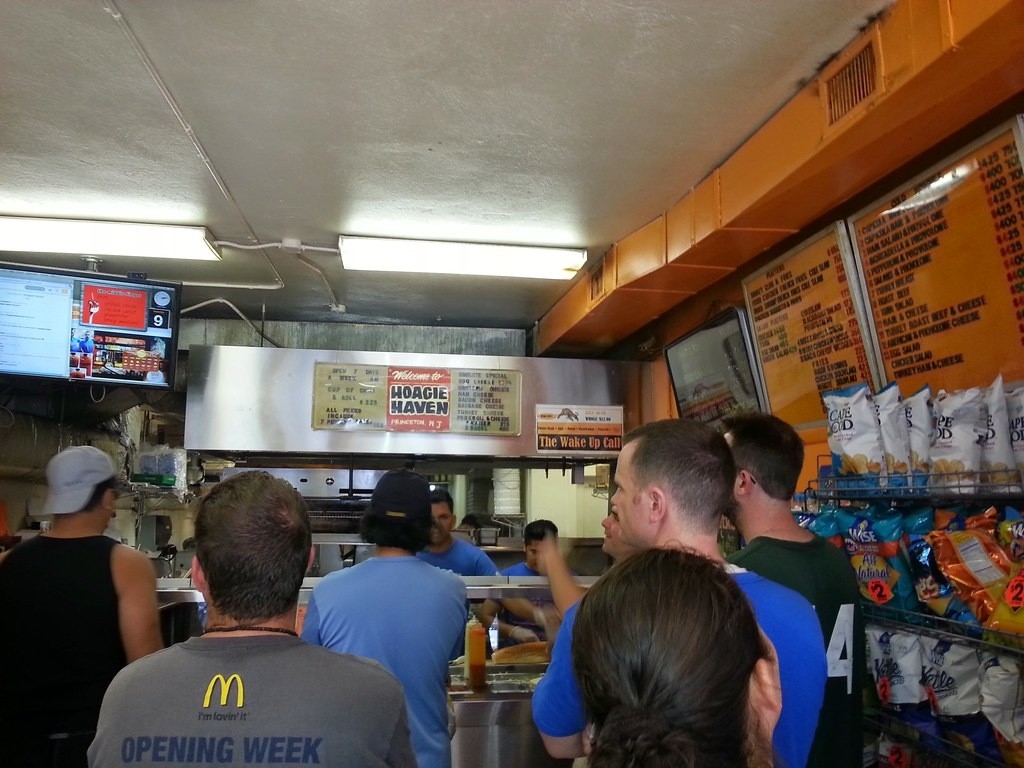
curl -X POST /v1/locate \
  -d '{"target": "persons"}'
[690,407,870,768]
[78,329,94,353]
[293,466,466,768]
[524,412,831,768]
[571,538,784,768]
[416,480,558,676]
[478,510,579,653]
[82,469,421,768]
[70,326,82,353]
[0,438,167,768]
[597,496,646,565]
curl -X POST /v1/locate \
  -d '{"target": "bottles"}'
[468,621,486,689]
[464,614,481,679]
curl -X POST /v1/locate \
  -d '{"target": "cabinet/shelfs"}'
[790,454,1024,767]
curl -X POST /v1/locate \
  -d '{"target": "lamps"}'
[337,235,589,282]
[0,213,224,265]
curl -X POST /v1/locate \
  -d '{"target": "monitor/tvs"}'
[0,260,183,390]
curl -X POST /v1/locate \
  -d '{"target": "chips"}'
[795,376,1024,768]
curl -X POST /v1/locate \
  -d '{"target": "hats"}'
[369,467,427,519]
[38,445,114,513]
[426,482,453,505]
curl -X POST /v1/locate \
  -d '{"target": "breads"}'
[491,642,550,664]
